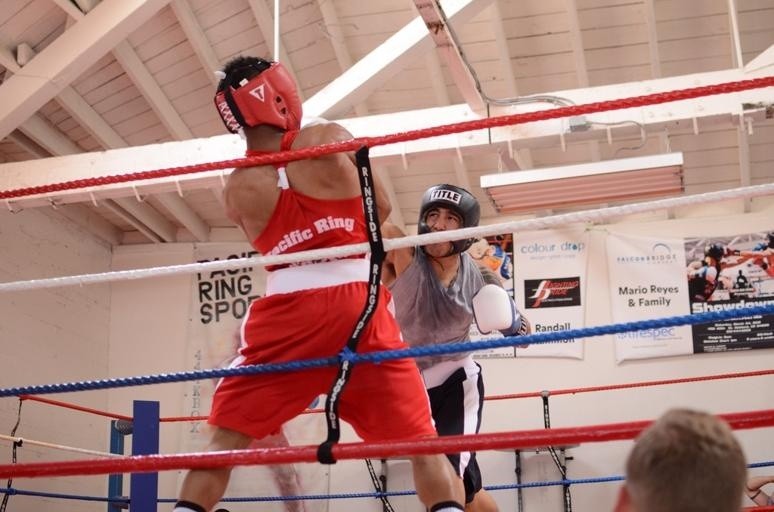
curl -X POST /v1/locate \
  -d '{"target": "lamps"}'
[479,151,686,216]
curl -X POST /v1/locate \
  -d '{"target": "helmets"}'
[214,62,302,135]
[416,183,479,256]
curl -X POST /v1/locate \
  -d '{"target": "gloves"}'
[471,284,527,337]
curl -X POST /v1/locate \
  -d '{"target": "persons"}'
[212,327,319,511]
[722,231,774,277]
[746,475,774,507]
[301,115,532,512]
[611,407,746,510]
[172,54,467,512]
[687,241,769,302]
